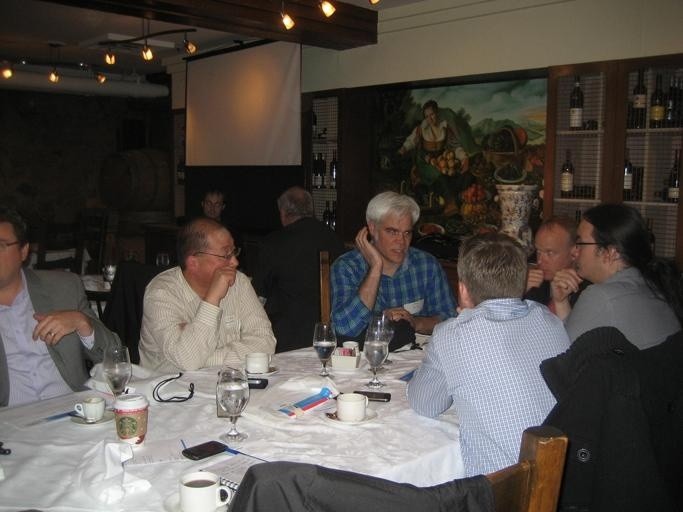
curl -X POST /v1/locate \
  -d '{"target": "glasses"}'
[194,246,242,260]
[1,240,19,255]
[574,238,597,249]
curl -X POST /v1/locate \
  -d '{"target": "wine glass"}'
[217,369,248,443]
[364,329,389,390]
[313,322,340,381]
[362,312,394,366]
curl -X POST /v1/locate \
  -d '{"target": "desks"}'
[81,261,185,291]
[1,333,459,512]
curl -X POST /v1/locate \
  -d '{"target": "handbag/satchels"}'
[336,320,414,354]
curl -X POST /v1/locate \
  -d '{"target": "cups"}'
[336,392,371,423]
[103,263,115,283]
[343,339,360,357]
[74,397,105,423]
[245,353,275,374]
[177,470,231,512]
[115,394,148,446]
[156,251,170,268]
[100,345,132,394]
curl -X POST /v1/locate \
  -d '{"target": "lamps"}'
[97,74,105,83]
[279,12,294,31]
[105,52,115,65]
[49,71,58,83]
[317,1,335,17]
[141,45,153,60]
[2,64,14,80]
[181,39,195,55]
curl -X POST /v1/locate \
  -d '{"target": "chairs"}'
[144,221,178,265]
[103,260,154,364]
[74,238,110,319]
[589,329,683,512]
[249,427,568,512]
[319,251,335,339]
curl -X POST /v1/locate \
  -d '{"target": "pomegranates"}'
[461,183,486,202]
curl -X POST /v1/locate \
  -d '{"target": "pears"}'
[439,195,445,205]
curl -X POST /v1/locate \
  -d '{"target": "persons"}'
[260,185,346,336]
[138,215,278,372]
[391,98,478,189]
[327,188,457,344]
[522,213,588,318]
[200,188,225,222]
[561,204,681,351]
[0,206,120,411]
[404,231,571,472]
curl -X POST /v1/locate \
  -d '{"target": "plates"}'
[319,409,380,428]
[161,492,230,512]
[71,408,113,426]
[495,164,528,184]
[417,223,444,238]
[246,367,280,378]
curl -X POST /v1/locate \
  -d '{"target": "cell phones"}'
[182,441,228,460]
[353,390,390,402]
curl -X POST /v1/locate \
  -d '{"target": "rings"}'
[48,331,55,336]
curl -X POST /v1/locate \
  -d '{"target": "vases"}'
[496,184,536,257]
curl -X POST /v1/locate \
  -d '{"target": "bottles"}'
[624,67,681,204]
[587,120,597,130]
[311,105,316,141]
[568,76,585,131]
[574,185,595,197]
[560,148,575,197]
[312,147,338,231]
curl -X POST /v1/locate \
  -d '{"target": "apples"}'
[425,149,457,177]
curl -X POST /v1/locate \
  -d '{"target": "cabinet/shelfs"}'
[308,95,340,234]
[543,54,682,270]
